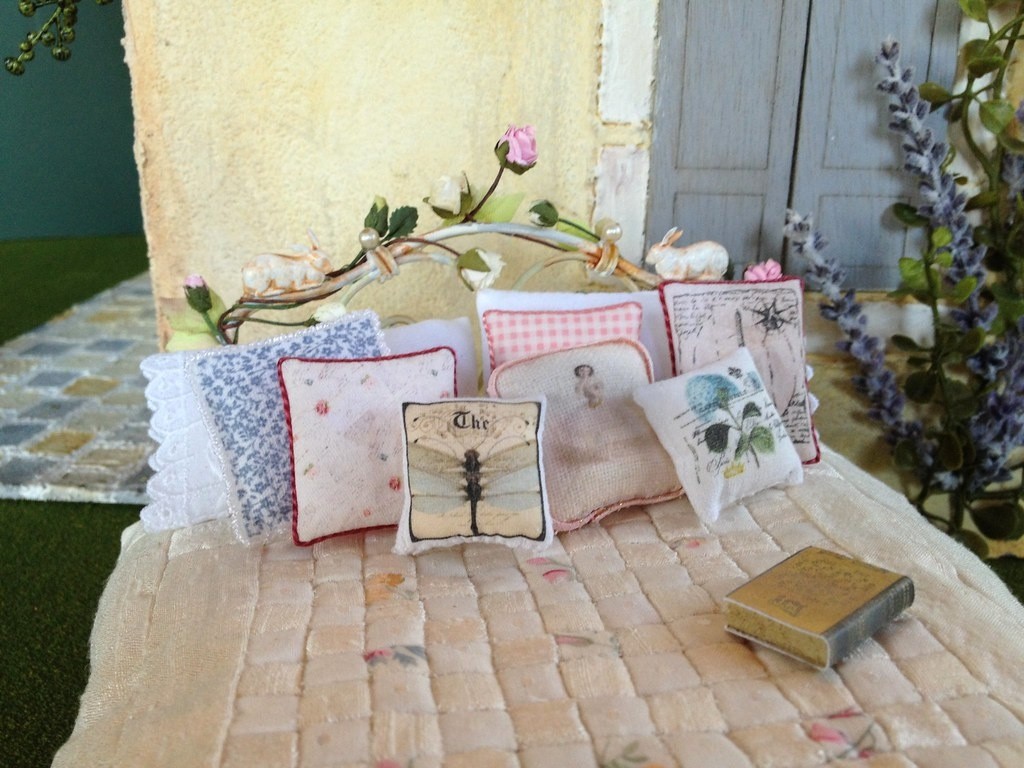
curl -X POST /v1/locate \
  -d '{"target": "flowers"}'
[180,124,782,348]
[182,125,540,348]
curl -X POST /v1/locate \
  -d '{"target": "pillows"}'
[391,394,554,556]
[482,301,643,374]
[142,316,477,534]
[278,346,459,547]
[488,338,684,531]
[632,347,803,525]
[659,276,820,464]
[184,310,391,546]
[476,289,819,443]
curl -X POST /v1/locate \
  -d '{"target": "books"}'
[724,545,915,671]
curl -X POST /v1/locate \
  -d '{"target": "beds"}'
[50,222,1024,768]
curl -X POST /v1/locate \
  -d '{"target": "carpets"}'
[0,265,159,505]
